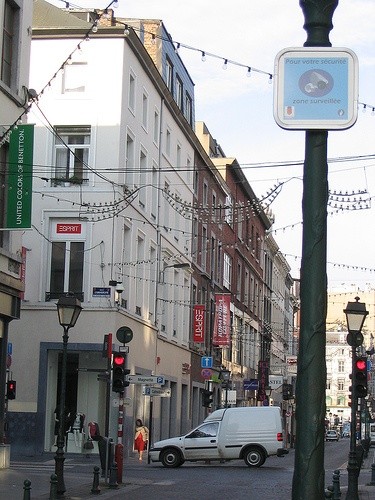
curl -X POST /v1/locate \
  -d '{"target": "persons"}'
[134,419,150,462]
[87,419,97,441]
[53,401,84,447]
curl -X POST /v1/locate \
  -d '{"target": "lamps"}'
[159,263,190,284]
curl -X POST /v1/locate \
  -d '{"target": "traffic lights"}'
[7,380,16,401]
[348,374,352,406]
[356,356,367,398]
[112,353,130,394]
[203,390,213,406]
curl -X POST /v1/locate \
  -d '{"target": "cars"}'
[325,430,339,442]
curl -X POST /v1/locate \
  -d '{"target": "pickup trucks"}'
[148,407,289,468]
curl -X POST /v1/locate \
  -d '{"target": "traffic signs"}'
[125,374,164,385]
[144,387,171,398]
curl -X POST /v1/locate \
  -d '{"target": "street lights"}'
[342,295,369,500]
[329,412,332,430]
[54,290,84,500]
